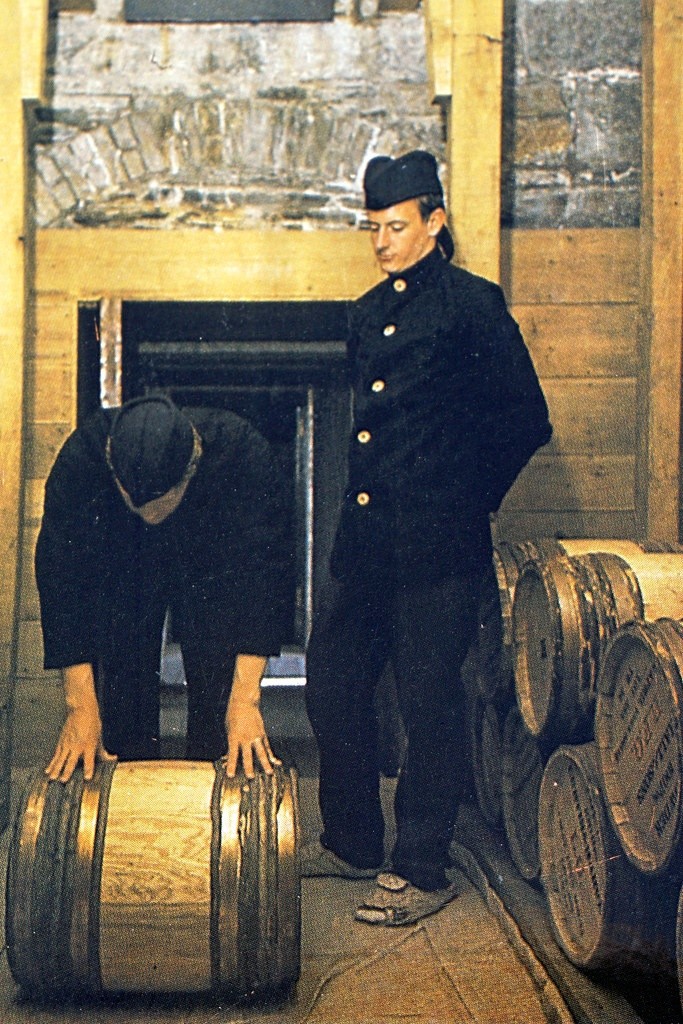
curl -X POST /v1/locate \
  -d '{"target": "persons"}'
[34,393,295,783]
[299,150,554,923]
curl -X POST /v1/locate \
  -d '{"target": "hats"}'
[362,151,444,209]
[108,393,193,508]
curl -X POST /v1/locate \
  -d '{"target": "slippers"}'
[297,831,384,879]
[354,871,460,928]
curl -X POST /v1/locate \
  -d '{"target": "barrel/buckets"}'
[6,761,302,999]
[470,536,683,987]
